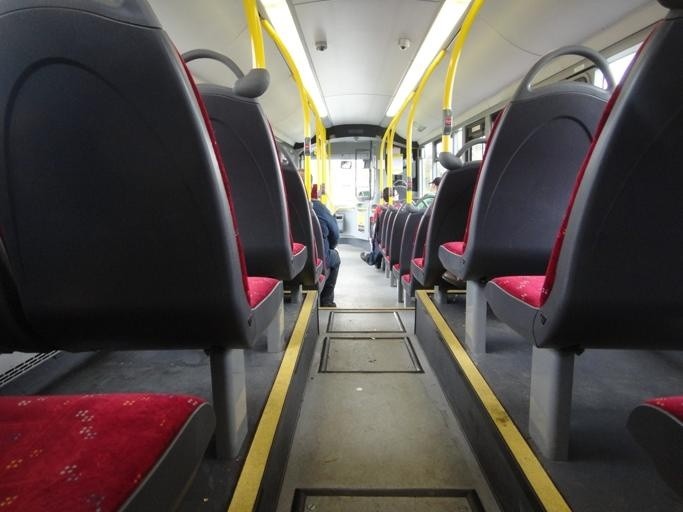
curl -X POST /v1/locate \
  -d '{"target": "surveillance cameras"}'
[396,39,411,50]
[312,41,327,53]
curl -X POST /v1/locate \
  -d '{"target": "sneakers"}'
[360,251,368,261]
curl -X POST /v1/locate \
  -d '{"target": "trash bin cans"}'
[335,213,344,232]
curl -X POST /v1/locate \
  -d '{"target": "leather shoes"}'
[320,301,336,307]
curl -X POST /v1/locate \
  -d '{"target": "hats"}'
[428,177,441,185]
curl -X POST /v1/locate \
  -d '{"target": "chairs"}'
[369,1,682,512]
[1,0,333,510]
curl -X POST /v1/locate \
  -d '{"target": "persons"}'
[360,176,443,270]
[296,168,341,307]
